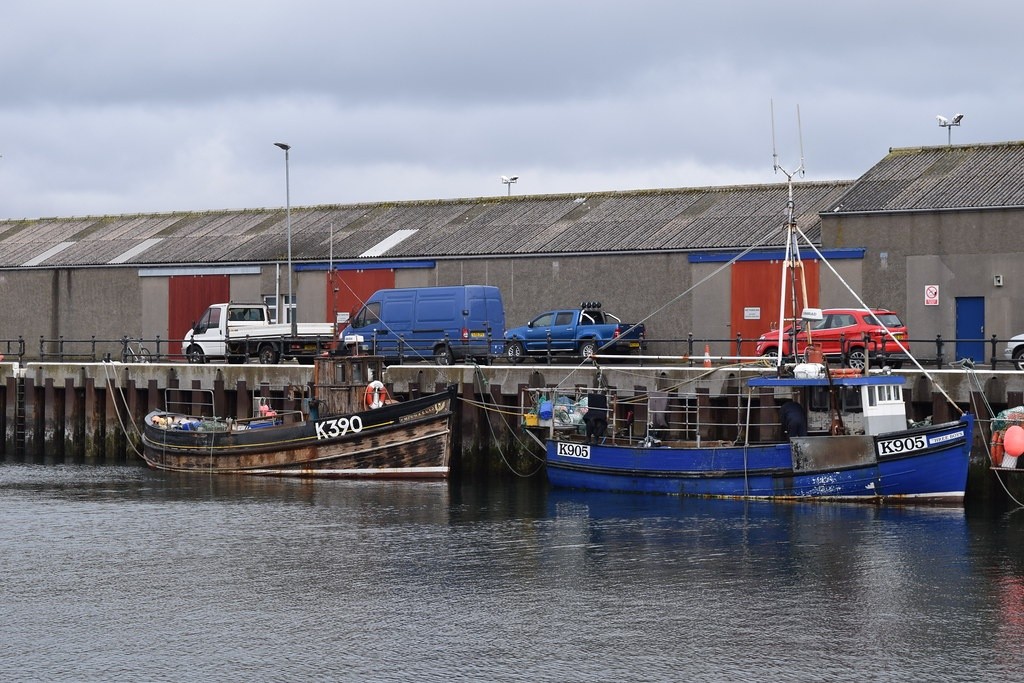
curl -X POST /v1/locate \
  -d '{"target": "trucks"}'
[180,300,338,364]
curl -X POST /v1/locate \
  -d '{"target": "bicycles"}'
[120,337,152,364]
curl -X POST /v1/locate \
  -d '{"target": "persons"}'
[779,401,808,442]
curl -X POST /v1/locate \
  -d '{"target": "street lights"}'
[500,175,518,196]
[274,141,294,323]
[935,112,964,146]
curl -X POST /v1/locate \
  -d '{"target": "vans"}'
[337,284,505,367]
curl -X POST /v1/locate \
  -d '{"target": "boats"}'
[137,221,466,486]
[516,99,977,511]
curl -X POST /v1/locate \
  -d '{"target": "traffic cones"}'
[702,344,712,367]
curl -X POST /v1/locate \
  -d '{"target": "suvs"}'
[756,307,911,374]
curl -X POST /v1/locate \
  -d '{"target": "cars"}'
[1005,333,1024,371]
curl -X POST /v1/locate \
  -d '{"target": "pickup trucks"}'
[503,300,647,364]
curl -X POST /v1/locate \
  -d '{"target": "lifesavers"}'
[828,368,862,379]
[366,380,387,409]
[996,434,1004,467]
[991,431,999,468]
